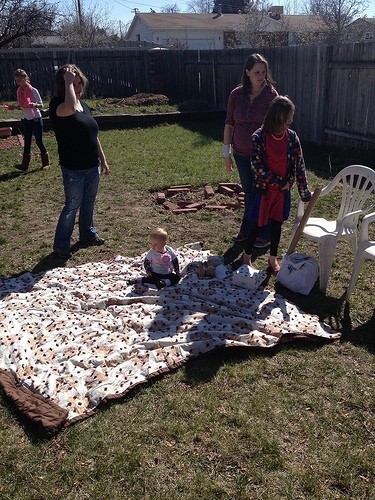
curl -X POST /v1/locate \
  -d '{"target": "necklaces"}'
[271,130,286,141]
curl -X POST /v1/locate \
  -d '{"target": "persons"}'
[241,96,311,272]
[7,68,49,171]
[222,52,279,247]
[49,64,111,260]
[132,227,181,288]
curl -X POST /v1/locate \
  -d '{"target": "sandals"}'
[268,258,281,272]
[241,253,252,266]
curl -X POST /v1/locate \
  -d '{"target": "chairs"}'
[290,165,375,291]
[349,212,375,290]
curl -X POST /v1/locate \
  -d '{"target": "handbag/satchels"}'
[229,263,268,290]
[275,253,318,295]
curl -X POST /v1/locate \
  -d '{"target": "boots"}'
[13,153,31,170]
[40,152,50,170]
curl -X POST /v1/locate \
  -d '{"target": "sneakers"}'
[253,239,271,248]
[232,232,247,241]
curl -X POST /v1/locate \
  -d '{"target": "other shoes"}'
[79,232,105,245]
[53,246,72,258]
[156,279,166,289]
[127,278,137,284]
